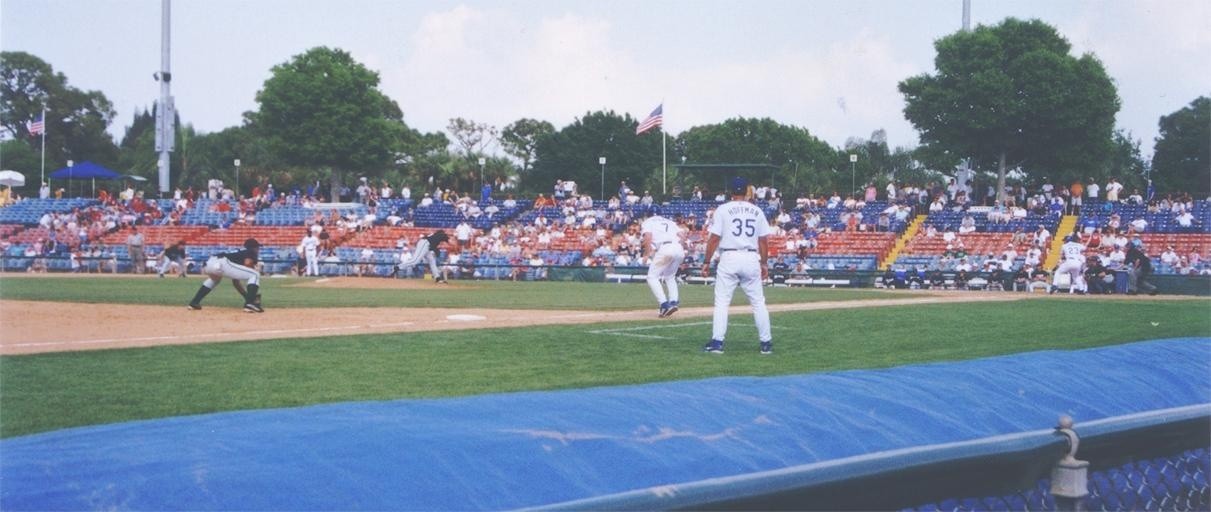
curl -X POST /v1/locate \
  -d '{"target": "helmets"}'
[732,176,746,195]
[648,204,662,217]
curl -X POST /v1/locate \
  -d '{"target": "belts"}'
[724,248,757,252]
[663,240,672,244]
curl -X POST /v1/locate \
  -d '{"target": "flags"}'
[30,109,43,137]
[636,104,663,135]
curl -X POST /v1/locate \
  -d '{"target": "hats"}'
[244,239,263,247]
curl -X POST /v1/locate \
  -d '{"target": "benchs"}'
[686,277,717,283]
[784,278,851,287]
[606,272,649,281]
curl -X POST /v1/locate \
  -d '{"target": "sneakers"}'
[188,303,202,309]
[437,277,447,284]
[760,342,773,354]
[660,301,679,317]
[1050,286,1055,293]
[244,302,264,312]
[701,340,724,353]
[390,265,399,277]
